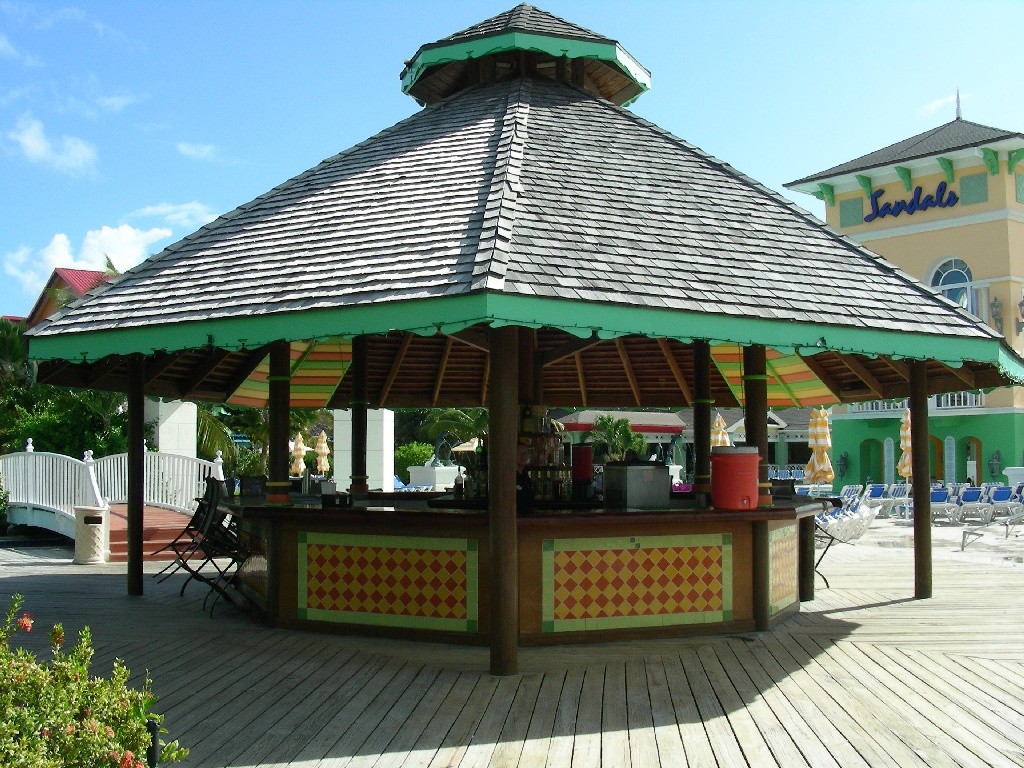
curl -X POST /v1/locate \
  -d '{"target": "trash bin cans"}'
[73,506,107,565]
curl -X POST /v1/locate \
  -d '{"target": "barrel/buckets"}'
[710,446,762,509]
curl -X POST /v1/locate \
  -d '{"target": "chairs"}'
[772,470,1024,548]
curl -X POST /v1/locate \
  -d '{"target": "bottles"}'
[455,473,471,495]
[476,440,482,453]
[525,470,572,500]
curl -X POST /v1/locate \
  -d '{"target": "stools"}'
[814,520,857,593]
[151,476,258,620]
